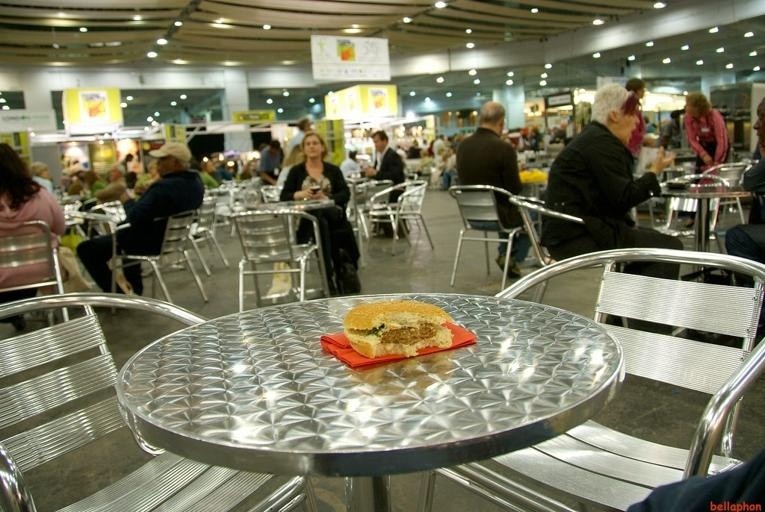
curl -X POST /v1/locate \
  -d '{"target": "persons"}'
[455,101,538,279]
[724,96,765,338]
[518,115,569,151]
[625,78,731,251]
[2,119,457,331]
[538,81,684,333]
[626,447,765,511]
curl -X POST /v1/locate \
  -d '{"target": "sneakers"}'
[495,252,521,278]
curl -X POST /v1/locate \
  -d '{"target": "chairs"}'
[0,291,321,512]
[366,180,435,256]
[86,200,123,239]
[261,185,284,203]
[188,197,230,276]
[507,195,630,328]
[356,180,399,241]
[212,188,246,236]
[701,162,753,225]
[0,220,70,322]
[193,189,215,236]
[228,209,331,312]
[110,209,209,304]
[425,247,765,512]
[659,173,731,255]
[449,184,538,291]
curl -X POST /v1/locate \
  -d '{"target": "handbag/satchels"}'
[339,248,360,292]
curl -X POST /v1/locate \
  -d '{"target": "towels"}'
[320,322,477,369]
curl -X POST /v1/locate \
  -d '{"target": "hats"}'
[149,142,191,162]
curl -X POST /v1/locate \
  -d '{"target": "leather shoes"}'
[328,277,337,294]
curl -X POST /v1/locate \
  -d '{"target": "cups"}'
[310,182,321,194]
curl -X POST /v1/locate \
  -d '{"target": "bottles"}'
[359,165,366,177]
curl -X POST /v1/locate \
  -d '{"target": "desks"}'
[246,199,335,302]
[655,186,755,282]
[346,178,365,230]
[116,292,626,512]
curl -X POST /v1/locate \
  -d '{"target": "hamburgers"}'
[343,298,456,361]
